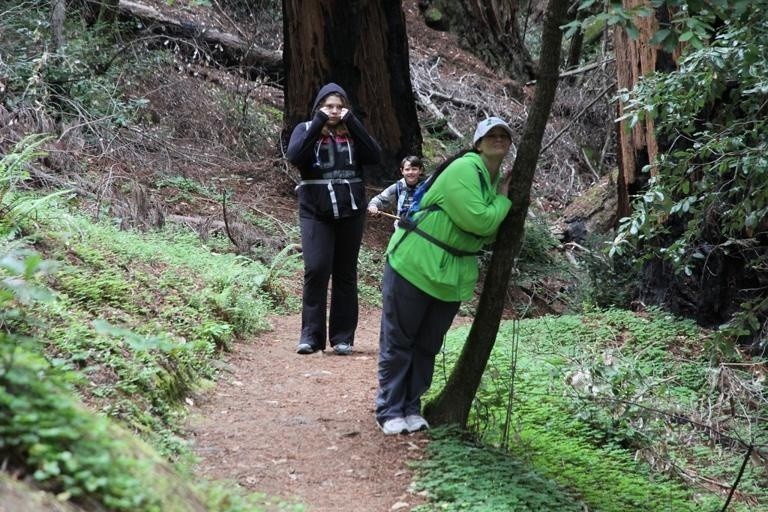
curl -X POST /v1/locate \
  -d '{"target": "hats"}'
[473,117,511,145]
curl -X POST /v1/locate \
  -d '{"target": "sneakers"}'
[376,413,430,434]
[297,342,353,354]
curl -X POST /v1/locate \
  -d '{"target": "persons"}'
[368,156,423,231]
[375,117,513,436]
[285,82,382,355]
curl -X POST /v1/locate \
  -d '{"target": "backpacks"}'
[405,175,433,217]
